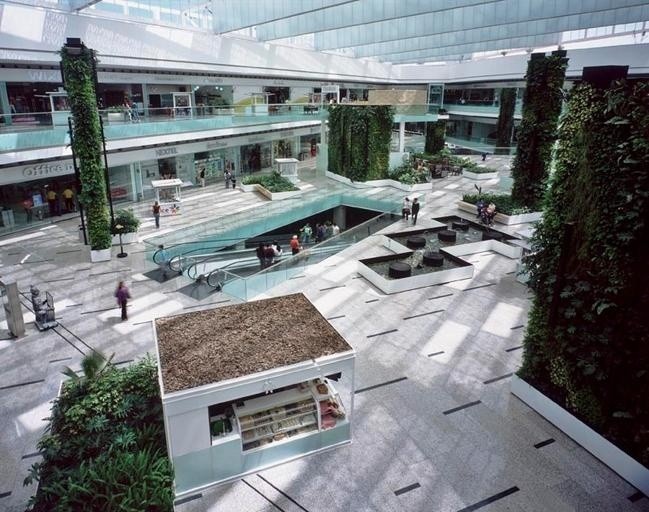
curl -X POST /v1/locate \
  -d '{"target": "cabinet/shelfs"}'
[231,387,318,444]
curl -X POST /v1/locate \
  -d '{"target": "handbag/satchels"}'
[404,208,410,215]
[262,258,270,268]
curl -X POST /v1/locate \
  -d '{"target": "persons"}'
[114,282,129,321]
[62,186,74,210]
[158,245,168,282]
[224,171,232,188]
[412,198,419,225]
[231,176,237,189]
[152,201,160,229]
[477,198,496,224]
[47,188,57,216]
[403,198,410,220]
[200,168,205,187]
[122,98,131,122]
[31,284,47,322]
[23,196,33,224]
[131,101,140,122]
[256,222,340,273]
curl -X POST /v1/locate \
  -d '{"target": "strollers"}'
[479,208,497,227]
